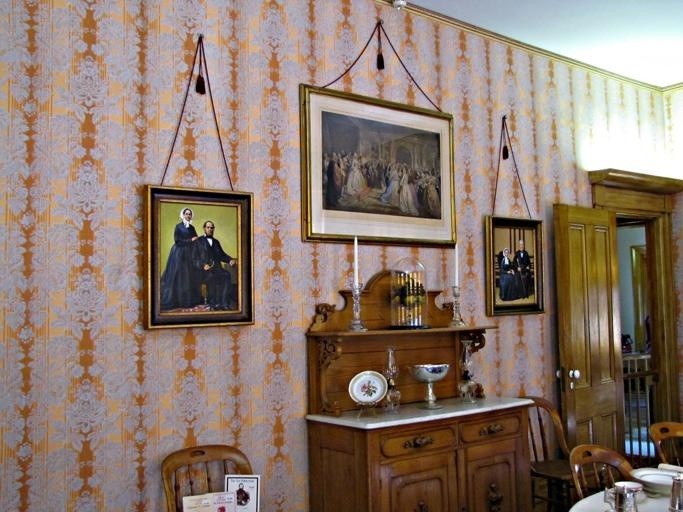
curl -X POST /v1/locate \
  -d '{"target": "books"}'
[208,491,238,512]
[182,492,214,512]
[225,473,260,512]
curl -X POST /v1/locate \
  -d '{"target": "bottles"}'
[456,338,479,404]
[667,470,683,512]
[383,347,402,413]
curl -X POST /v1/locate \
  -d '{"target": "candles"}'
[452,240,461,287]
[352,234,360,289]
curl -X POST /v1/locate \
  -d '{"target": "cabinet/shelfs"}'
[307,269,534,512]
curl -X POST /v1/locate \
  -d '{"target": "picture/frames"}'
[298,84,458,250]
[143,181,257,328]
[483,214,545,318]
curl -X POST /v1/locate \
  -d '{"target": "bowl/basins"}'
[630,467,683,496]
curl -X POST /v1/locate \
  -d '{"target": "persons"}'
[322,146,441,220]
[498,247,523,301]
[512,240,534,298]
[217,507,225,512]
[234,482,250,507]
[190,220,237,311]
[158,207,206,312]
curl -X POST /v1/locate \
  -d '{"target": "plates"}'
[347,370,388,405]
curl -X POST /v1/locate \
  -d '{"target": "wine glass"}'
[408,362,450,410]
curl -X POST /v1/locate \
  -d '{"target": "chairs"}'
[517,396,683,512]
[161,445,254,512]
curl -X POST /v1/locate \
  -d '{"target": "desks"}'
[623,352,652,391]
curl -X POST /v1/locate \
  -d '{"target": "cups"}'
[602,480,643,512]
